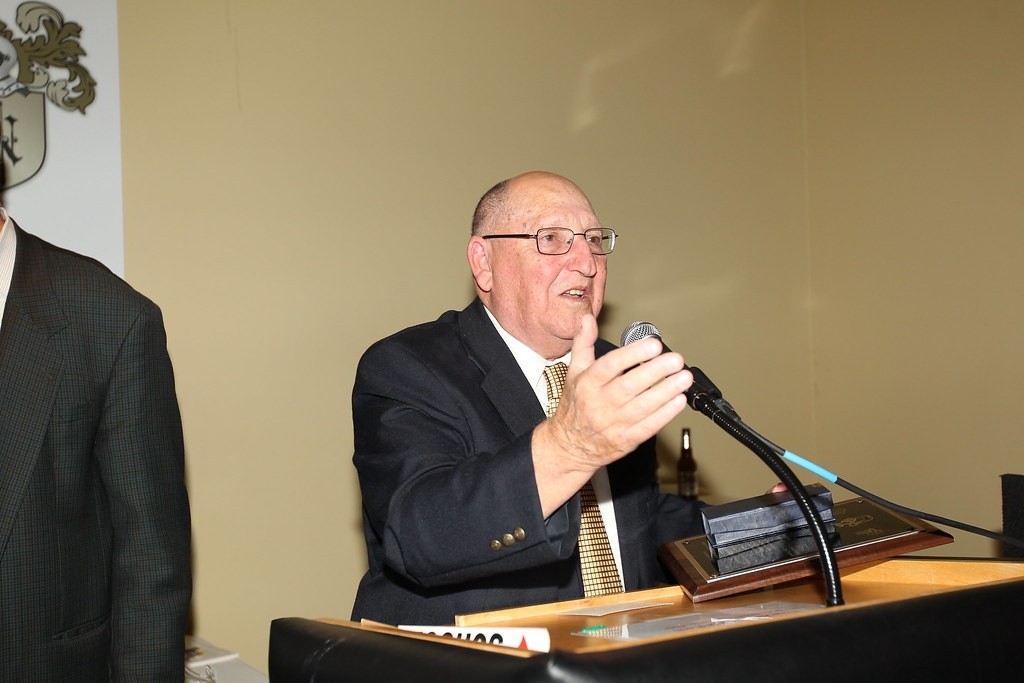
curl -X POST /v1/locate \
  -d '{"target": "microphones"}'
[621,319,711,412]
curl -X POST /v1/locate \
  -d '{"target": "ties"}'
[543,364,624,601]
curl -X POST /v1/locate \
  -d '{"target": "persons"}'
[351,171,789,628]
[0,103,194,683]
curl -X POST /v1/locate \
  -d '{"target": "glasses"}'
[481,227,619,255]
